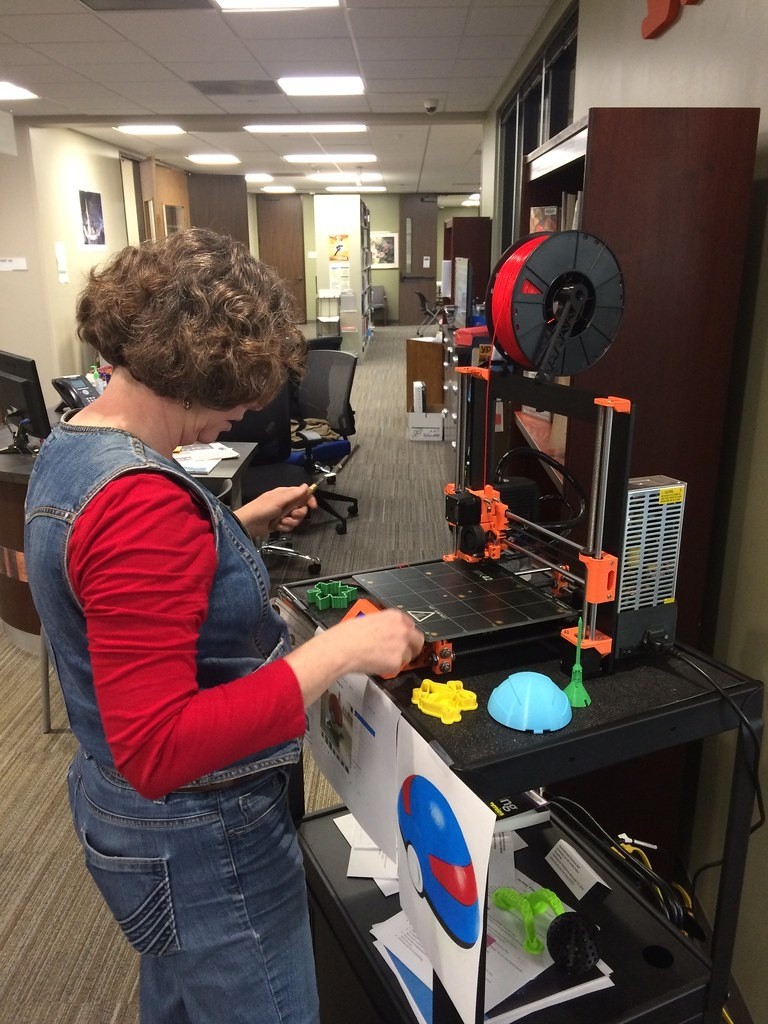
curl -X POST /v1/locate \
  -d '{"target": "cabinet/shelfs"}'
[315,297,340,336]
[442,217,493,305]
[405,338,444,413]
[276,561,764,1024]
[522,107,761,883]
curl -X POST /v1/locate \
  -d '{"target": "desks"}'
[0,410,260,655]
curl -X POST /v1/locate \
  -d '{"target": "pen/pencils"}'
[575,616,583,665]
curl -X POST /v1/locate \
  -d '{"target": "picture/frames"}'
[369,233,399,270]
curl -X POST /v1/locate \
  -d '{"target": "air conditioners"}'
[436,194,480,207]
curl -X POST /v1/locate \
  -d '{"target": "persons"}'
[23,226,425,1024]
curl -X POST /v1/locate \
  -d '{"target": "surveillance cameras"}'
[424,99,437,114]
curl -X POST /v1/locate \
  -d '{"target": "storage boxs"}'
[406,403,443,441]
[318,289,339,298]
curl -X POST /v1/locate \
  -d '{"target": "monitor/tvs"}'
[0,350,51,453]
[455,256,473,330]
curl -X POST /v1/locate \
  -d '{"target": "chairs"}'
[217,338,358,574]
[372,285,387,326]
[414,291,442,337]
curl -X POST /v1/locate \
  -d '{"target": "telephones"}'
[51,375,100,414]
